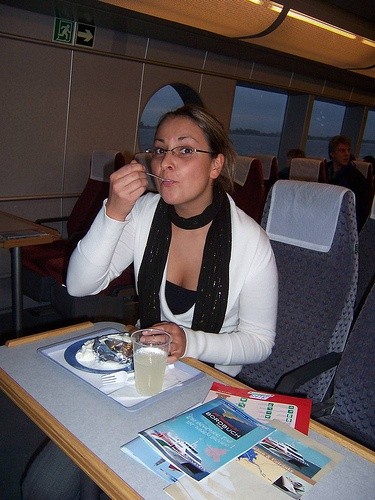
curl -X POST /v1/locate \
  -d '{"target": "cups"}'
[131,328,173,396]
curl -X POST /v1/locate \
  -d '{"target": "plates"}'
[64,332,135,375]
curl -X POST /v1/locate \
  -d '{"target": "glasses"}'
[145,145,215,159]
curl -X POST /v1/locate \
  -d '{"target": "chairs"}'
[12,150,375,451]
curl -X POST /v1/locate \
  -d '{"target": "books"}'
[119,394,321,500]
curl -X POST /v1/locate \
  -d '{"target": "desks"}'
[0,210,63,338]
[0,322,375,500]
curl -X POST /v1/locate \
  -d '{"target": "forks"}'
[101,364,175,385]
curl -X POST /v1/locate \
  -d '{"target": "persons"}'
[20,105,279,500]
[278,149,306,180]
[325,136,375,237]
